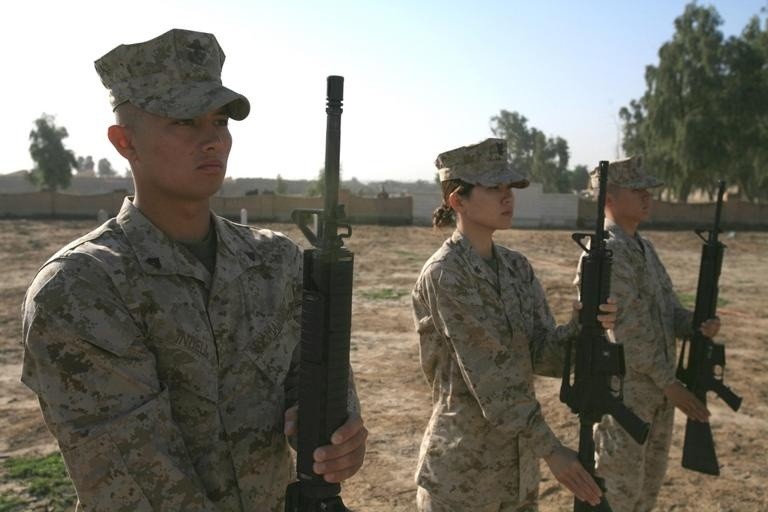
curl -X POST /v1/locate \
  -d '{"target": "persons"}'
[409,137,620,512]
[571,155,723,512]
[19,27,369,512]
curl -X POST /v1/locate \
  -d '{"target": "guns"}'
[559,160,650,511]
[286,75,354,512]
[676,178,743,476]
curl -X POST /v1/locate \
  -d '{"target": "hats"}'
[588,153,664,193]
[93,28,250,121]
[435,138,530,190]
